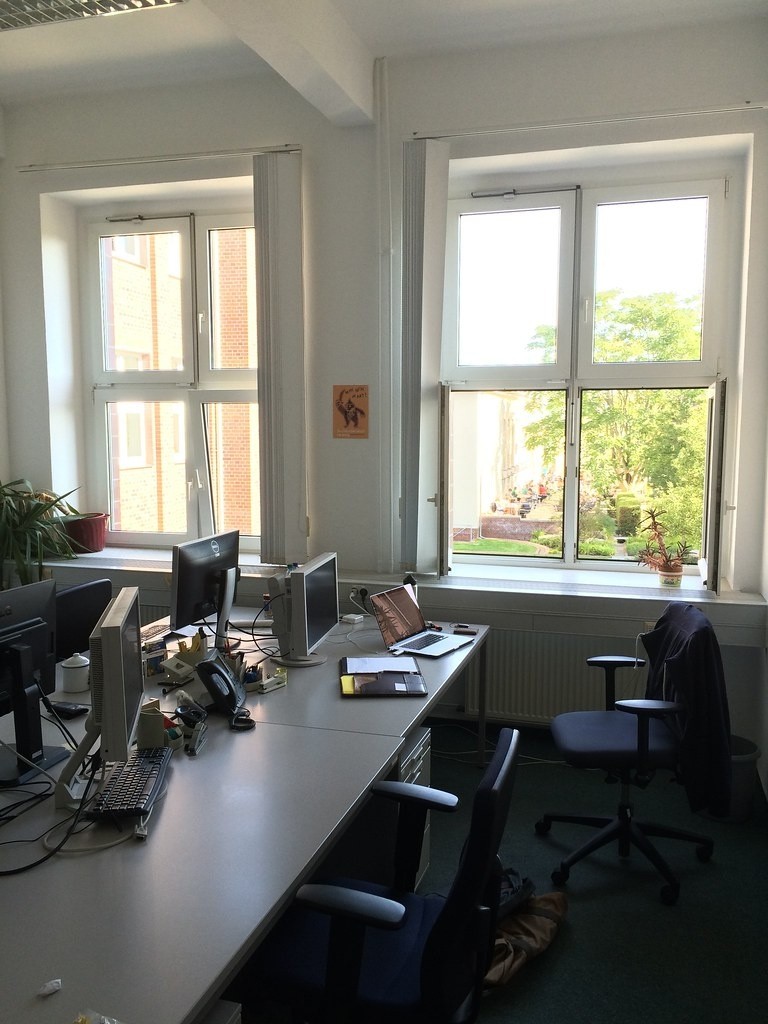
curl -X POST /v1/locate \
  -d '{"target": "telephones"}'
[194,648,256,731]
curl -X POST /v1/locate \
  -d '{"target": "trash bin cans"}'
[698,734,762,824]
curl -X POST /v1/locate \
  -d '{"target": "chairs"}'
[533,605,732,906]
[53,578,112,665]
[218,727,520,1024]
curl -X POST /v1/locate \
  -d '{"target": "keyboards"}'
[86,746,174,820]
[141,624,171,642]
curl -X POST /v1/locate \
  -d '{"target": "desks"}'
[0,605,491,1024]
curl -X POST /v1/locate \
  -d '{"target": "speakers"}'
[175,705,207,728]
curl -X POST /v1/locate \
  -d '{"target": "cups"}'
[60,653,90,693]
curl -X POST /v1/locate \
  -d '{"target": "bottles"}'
[286,562,300,577]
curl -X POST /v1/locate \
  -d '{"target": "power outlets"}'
[351,585,369,598]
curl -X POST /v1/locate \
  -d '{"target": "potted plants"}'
[637,507,692,587]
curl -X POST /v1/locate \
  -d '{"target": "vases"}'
[64,512,111,554]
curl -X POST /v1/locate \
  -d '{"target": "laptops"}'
[370,583,475,659]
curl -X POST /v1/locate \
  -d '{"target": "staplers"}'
[258,677,287,694]
[184,723,208,758]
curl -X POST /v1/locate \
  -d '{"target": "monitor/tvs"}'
[270,552,339,668]
[170,529,241,646]
[0,579,72,788]
[51,586,144,818]
